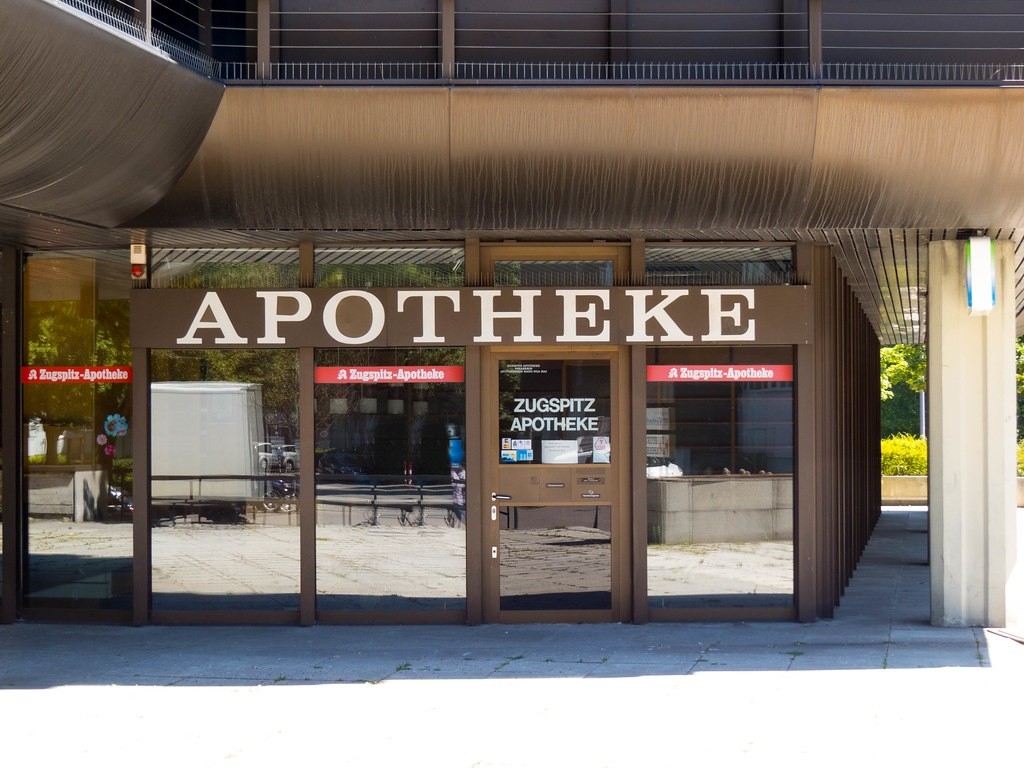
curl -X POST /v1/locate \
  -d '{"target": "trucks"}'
[150,380,263,521]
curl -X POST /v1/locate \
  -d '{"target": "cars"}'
[317,453,368,484]
[278,446,301,473]
[254,441,279,471]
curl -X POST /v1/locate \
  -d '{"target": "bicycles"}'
[260,479,300,514]
